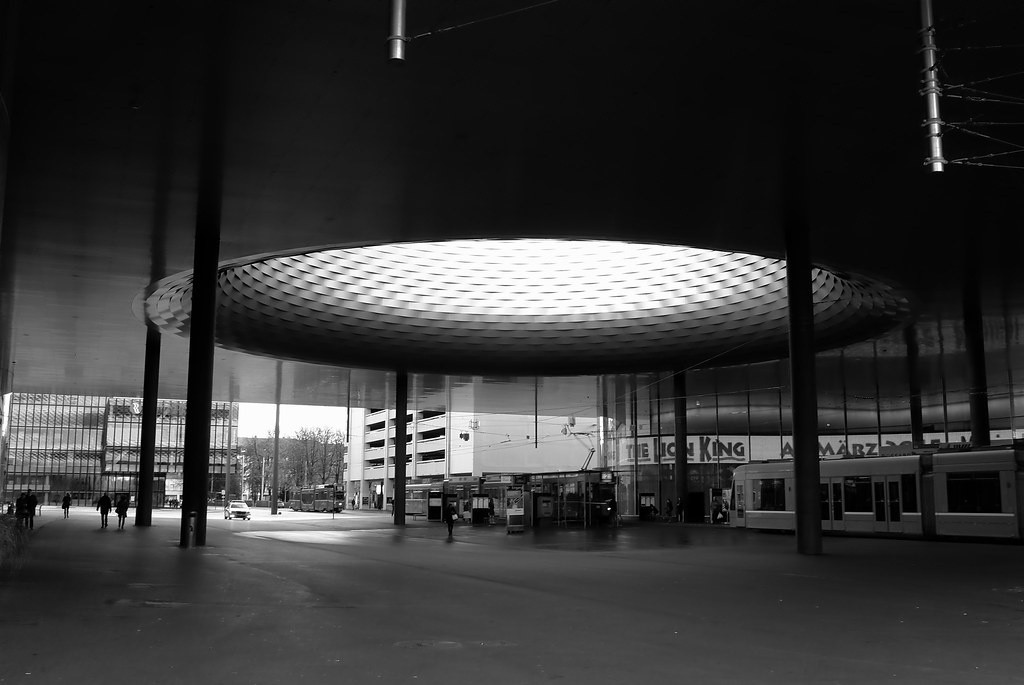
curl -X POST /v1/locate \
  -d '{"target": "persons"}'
[62,492,71,519]
[96,493,111,528]
[488,499,496,525]
[675,497,684,522]
[351,499,355,510]
[116,494,129,529]
[666,499,674,524]
[709,497,721,525]
[15,489,38,530]
[442,503,458,539]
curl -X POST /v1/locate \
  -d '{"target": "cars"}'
[224,500,251,520]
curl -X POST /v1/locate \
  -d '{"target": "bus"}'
[289,483,347,513]
[729,448,1024,543]
[405,483,443,515]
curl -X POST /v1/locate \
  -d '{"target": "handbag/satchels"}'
[452,514,458,520]
[716,512,723,519]
